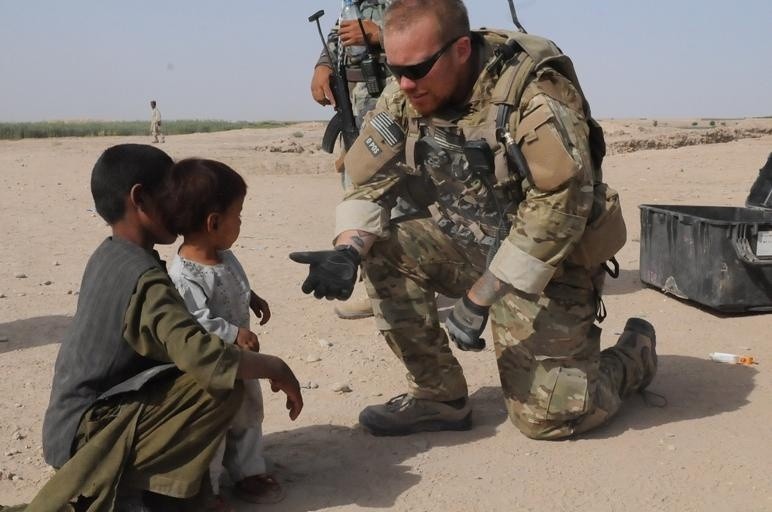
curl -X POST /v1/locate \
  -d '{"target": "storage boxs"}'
[638,152,772,314]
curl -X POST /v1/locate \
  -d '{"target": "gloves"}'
[444,291,490,352]
[289,244,365,301]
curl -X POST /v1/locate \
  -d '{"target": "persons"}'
[310,0,402,320]
[157,157,285,512]
[39,143,303,510]
[149,100,165,143]
[290,1,660,442]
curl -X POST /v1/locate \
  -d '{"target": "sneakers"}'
[360,391,473,435]
[616,315,668,411]
[140,472,286,511]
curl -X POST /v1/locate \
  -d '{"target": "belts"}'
[344,67,389,83]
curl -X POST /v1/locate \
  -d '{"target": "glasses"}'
[384,37,459,85]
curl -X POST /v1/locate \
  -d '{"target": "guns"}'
[321,72,360,152]
